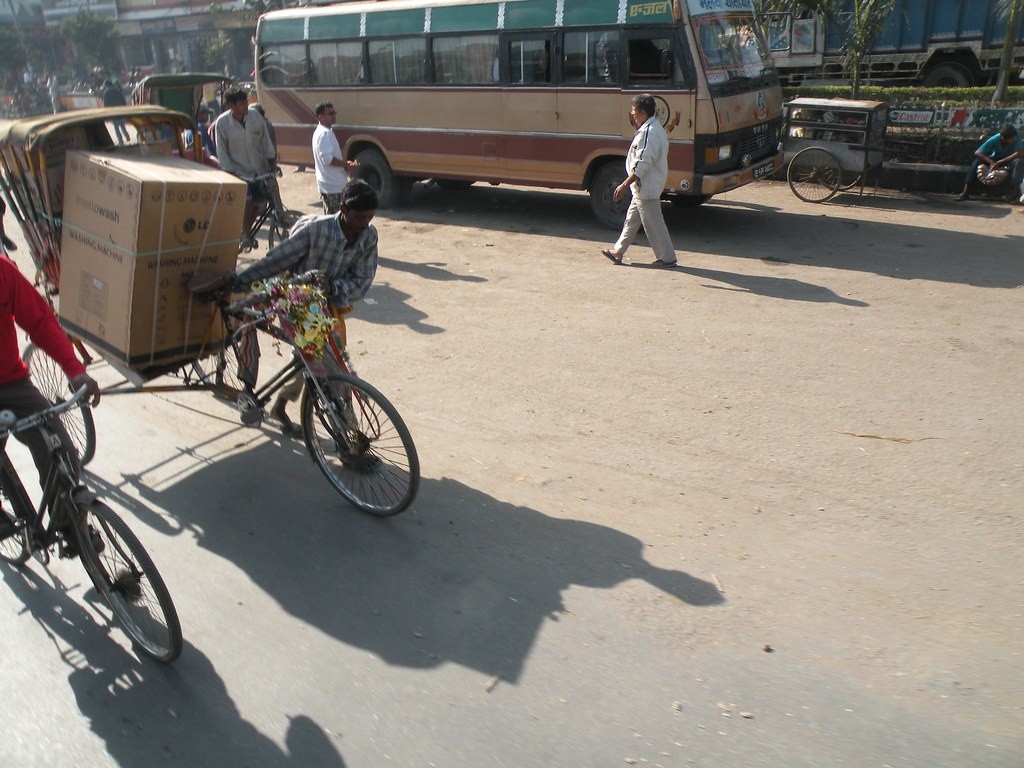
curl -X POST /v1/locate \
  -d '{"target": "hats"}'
[249,103,263,113]
[199,102,215,113]
[250,70,255,77]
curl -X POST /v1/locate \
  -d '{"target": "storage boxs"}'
[58,149,248,368]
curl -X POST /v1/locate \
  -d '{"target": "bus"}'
[250,0,787,234]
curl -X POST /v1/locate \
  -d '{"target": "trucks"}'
[740,0,1024,89]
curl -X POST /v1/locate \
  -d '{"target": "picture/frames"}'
[790,16,817,55]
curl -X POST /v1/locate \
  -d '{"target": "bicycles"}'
[0,383,183,665]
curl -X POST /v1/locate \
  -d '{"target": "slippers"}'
[601,249,622,264]
[342,452,381,472]
[280,422,306,440]
[651,260,678,268]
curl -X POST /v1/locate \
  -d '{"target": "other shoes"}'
[0,508,18,540]
[1011,197,1023,204]
[955,192,969,201]
[1002,195,1010,201]
[241,234,258,248]
[66,525,105,553]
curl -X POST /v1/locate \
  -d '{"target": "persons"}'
[128,66,141,92]
[223,179,382,472]
[602,94,678,266]
[73,72,130,146]
[0,254,106,553]
[186,104,217,158]
[4,69,61,118]
[215,90,280,248]
[223,76,241,111]
[955,124,1024,204]
[312,102,360,215]
[668,46,690,87]
[249,102,277,154]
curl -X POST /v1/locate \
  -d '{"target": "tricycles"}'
[131,71,234,163]
[0,103,420,518]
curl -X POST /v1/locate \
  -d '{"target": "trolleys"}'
[781,97,925,203]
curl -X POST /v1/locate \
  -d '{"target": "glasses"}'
[322,111,337,115]
